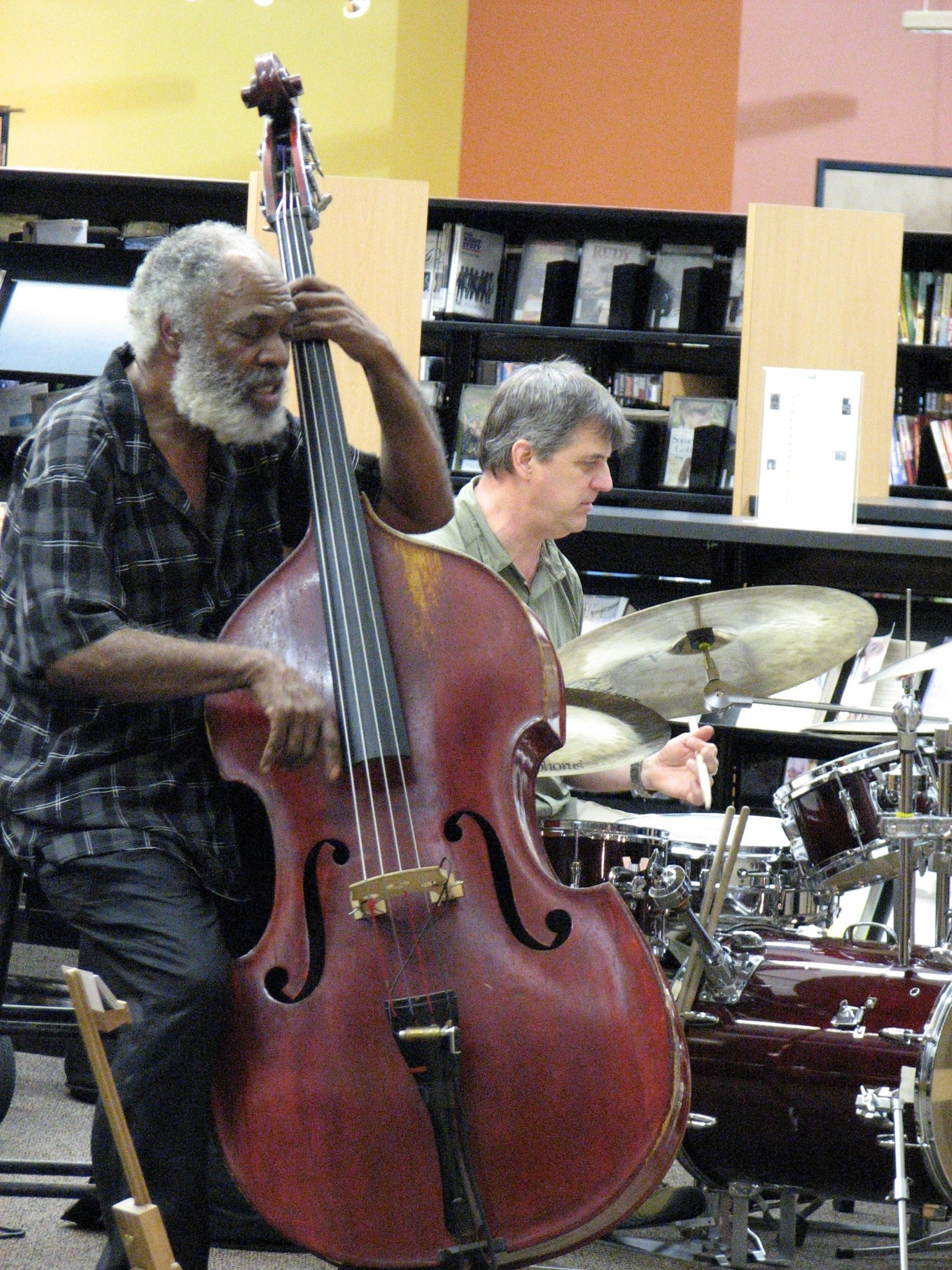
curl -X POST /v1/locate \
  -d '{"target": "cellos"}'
[194,55,688,1270]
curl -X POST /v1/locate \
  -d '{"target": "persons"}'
[455,265,495,305]
[405,358,718,1231]
[0,219,454,1270]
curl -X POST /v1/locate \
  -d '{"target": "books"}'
[889,270,952,489]
[421,225,743,333]
[428,359,734,493]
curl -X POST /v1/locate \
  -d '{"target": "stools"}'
[0,827,136,1205]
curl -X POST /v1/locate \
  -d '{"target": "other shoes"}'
[613,1180,707,1230]
[64,1044,113,1103]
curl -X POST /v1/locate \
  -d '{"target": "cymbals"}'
[554,586,880,720]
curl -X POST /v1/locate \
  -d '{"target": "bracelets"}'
[629,760,660,799]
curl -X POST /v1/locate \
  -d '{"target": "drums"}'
[538,817,671,957]
[670,837,836,931]
[770,740,949,895]
[670,925,949,1203]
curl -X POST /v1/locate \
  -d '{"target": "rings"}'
[710,767,718,776]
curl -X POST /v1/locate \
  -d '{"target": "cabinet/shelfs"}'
[419,195,902,517]
[1,165,426,502]
[893,229,952,503]
